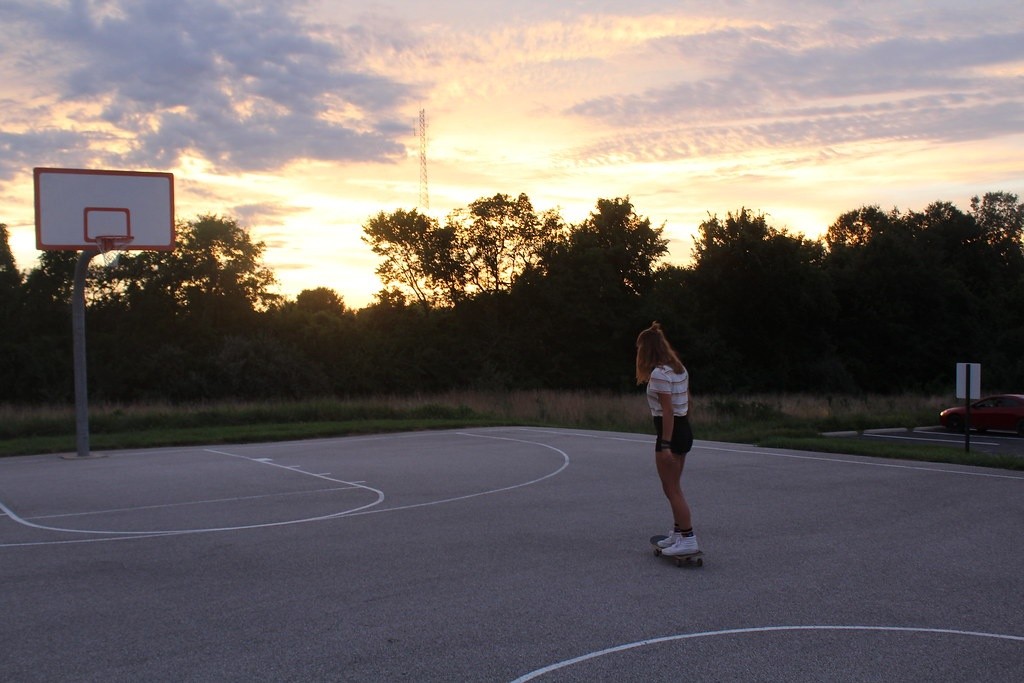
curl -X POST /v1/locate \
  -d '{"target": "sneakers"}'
[657,530,699,555]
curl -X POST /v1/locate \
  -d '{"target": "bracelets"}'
[660,439,672,450]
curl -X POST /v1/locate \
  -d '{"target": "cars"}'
[938,395,1024,435]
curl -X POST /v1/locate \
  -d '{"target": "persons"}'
[634,322,702,558]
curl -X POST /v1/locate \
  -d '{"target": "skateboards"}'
[650,535,704,568]
